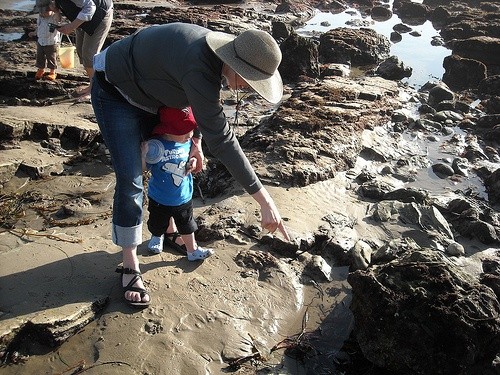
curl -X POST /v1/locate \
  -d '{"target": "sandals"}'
[187,247,214,261]
[115,266,151,305]
[164,231,187,256]
[147,234,164,253]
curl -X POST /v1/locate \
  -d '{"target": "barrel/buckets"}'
[58,33,76,69]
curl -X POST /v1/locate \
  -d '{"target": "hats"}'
[152,105,198,135]
[206,29,283,104]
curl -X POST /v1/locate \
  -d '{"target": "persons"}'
[90,21,292,306]
[144,104,214,262]
[28,0,113,95]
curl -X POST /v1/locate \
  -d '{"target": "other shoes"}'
[47,72,57,79]
[35,69,44,79]
